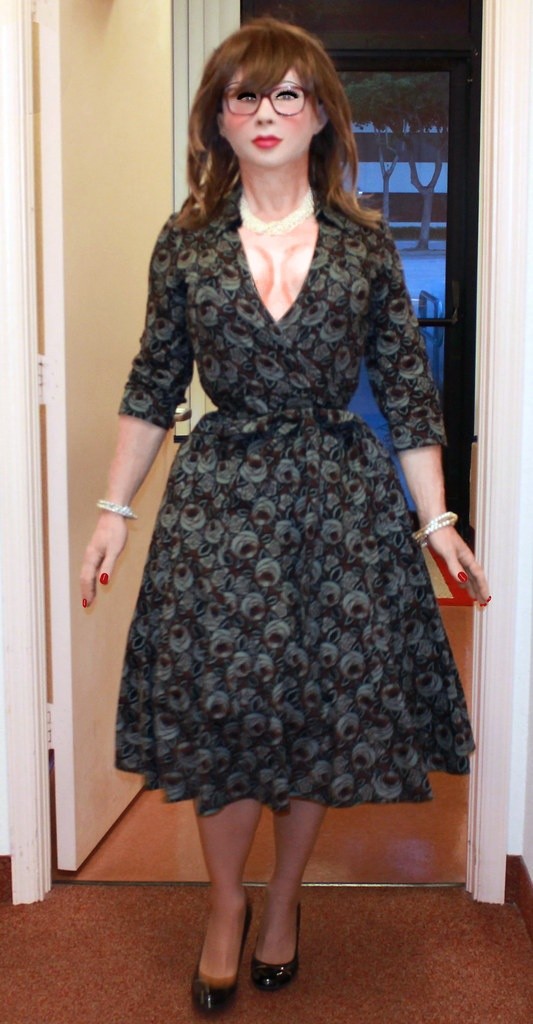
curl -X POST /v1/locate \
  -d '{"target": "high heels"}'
[250,902,302,991]
[192,902,253,1018]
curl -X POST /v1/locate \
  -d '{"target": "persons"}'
[78,19,490,1011]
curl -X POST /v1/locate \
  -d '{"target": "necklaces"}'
[239,191,316,237]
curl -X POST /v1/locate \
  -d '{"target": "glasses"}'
[223,80,313,117]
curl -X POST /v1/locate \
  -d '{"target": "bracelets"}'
[96,498,138,521]
[413,511,459,549]
